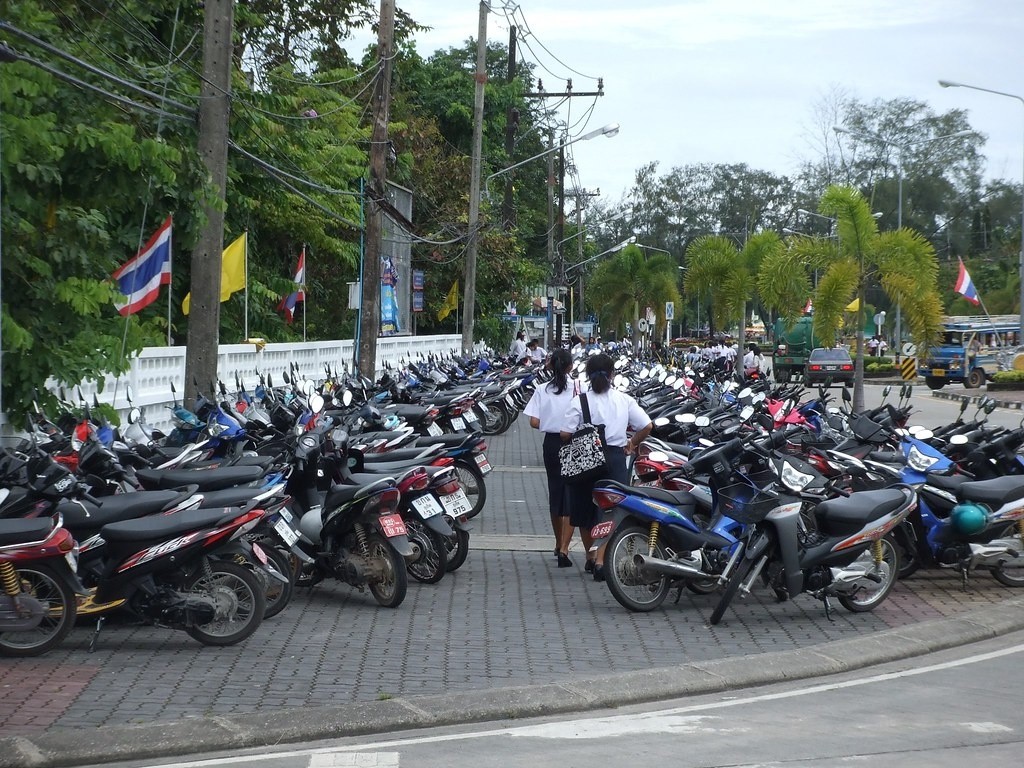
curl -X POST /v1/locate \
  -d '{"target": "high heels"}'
[554,547,560,556]
[593,564,605,582]
[585,558,595,573]
[558,552,572,567]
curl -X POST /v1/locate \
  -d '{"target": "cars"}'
[711,330,733,341]
[803,348,855,388]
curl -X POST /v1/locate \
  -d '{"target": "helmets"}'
[951,503,989,535]
[299,509,324,546]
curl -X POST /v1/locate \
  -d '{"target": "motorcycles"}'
[0,334,552,658]
[568,332,1024,613]
[709,412,919,625]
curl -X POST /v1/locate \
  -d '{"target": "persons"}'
[561,354,654,582]
[964,335,996,352]
[570,334,585,361]
[608,335,641,350]
[585,337,601,354]
[523,349,589,568]
[879,337,887,356]
[685,338,737,364]
[510,331,549,365]
[742,342,766,372]
[869,336,879,357]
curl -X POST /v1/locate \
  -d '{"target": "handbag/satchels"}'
[556,391,614,486]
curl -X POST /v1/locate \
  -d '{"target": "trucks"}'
[915,314,1023,390]
[766,316,837,386]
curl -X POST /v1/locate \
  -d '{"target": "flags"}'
[805,289,815,316]
[953,259,981,306]
[438,281,457,321]
[105,218,172,317]
[182,233,247,315]
[277,251,304,323]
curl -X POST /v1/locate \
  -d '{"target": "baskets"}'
[746,470,776,490]
[800,432,837,454]
[851,465,902,492]
[717,483,780,525]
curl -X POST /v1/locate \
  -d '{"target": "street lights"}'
[797,207,883,350]
[833,123,976,366]
[678,265,701,337]
[782,226,838,297]
